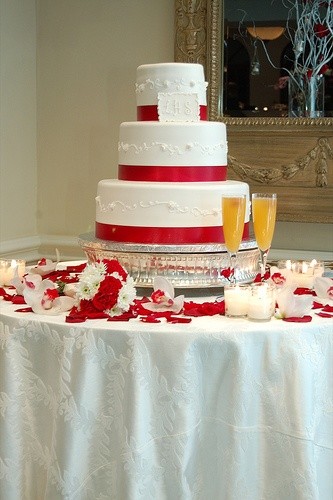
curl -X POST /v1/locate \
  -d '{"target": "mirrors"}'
[205,0,333,132]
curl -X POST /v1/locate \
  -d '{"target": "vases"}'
[287,75,324,117]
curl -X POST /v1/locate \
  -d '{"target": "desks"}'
[0,260,333,500]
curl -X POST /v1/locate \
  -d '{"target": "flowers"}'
[0,246,333,324]
[301,23,330,78]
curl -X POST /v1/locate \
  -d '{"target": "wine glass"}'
[221,194,247,285]
[252,193,277,283]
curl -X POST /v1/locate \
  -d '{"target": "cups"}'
[302,261,325,289]
[278,259,303,288]
[247,282,277,321]
[224,284,252,319]
[0,258,25,286]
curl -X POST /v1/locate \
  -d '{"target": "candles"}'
[248,282,275,321]
[278,259,297,271]
[224,281,252,318]
[0,259,25,283]
[302,258,325,276]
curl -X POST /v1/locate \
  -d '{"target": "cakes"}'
[95,62,251,245]
